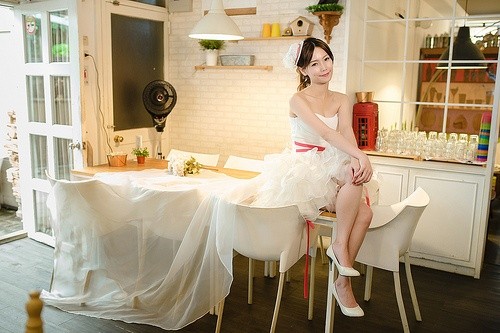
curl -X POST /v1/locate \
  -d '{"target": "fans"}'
[143,79,177,159]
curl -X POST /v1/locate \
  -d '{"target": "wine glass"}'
[450,86,458,102]
[435,93,442,105]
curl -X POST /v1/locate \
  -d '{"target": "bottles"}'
[425,32,450,48]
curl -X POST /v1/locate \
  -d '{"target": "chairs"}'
[44,149,429,333]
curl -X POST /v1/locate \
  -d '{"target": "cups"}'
[378,111,491,161]
[262,22,281,37]
[460,91,492,110]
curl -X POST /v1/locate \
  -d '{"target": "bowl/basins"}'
[220,54,254,66]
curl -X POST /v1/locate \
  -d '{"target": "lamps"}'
[190,0,244,40]
[436,0,489,70]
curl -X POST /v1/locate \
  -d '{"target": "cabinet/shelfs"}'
[364,156,484,274]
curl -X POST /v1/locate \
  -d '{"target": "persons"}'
[288,37,373,317]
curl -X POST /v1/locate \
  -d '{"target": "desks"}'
[72,159,380,333]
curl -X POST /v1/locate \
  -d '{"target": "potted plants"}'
[198,39,225,66]
[132,147,149,164]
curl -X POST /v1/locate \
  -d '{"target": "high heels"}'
[326,245,360,277]
[331,282,365,317]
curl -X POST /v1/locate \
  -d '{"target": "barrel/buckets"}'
[356,92,374,103]
[106,154,127,166]
